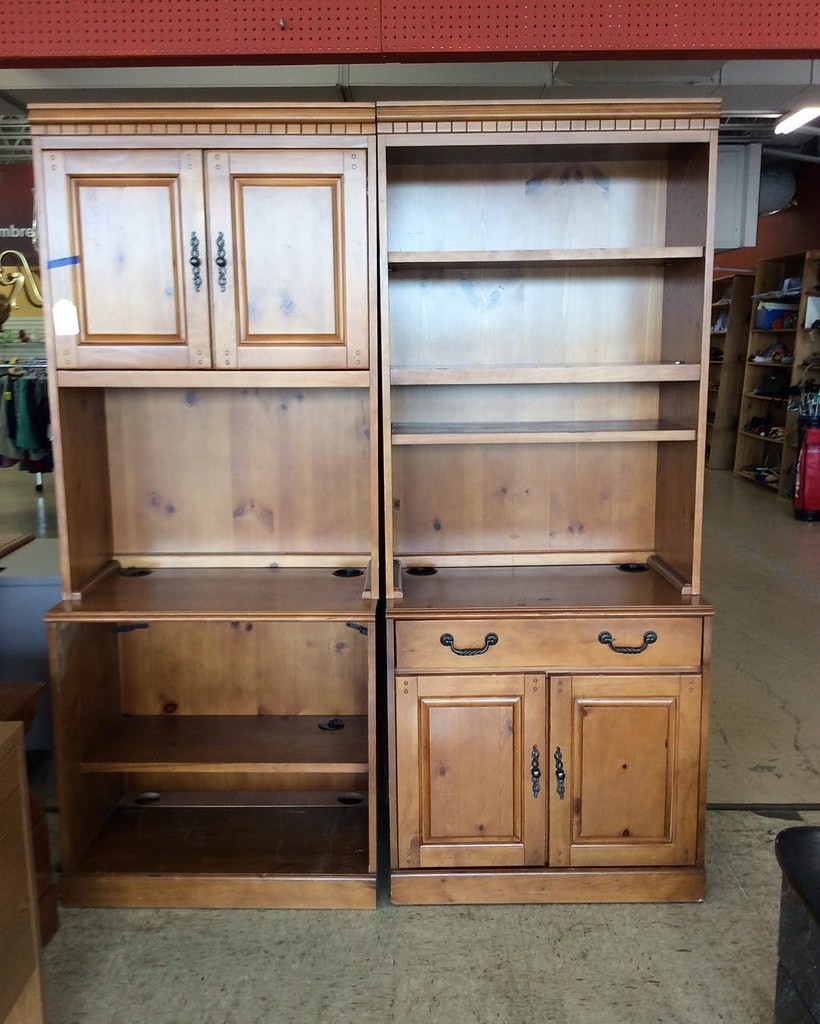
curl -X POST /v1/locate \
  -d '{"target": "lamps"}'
[774,101,820,135]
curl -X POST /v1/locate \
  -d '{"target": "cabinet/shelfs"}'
[377,98,723,903]
[27,99,382,910]
[733,248,820,499]
[705,274,756,470]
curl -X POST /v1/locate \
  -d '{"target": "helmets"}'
[753,343,789,364]
[752,367,791,395]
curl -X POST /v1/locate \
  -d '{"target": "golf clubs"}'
[786,392,820,427]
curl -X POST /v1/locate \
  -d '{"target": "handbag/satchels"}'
[755,300,799,330]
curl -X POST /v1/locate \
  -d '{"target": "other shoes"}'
[709,347,723,362]
[740,463,780,488]
[708,380,720,391]
[710,313,729,332]
[707,411,717,424]
[705,445,711,462]
[713,297,732,306]
[743,415,785,441]
